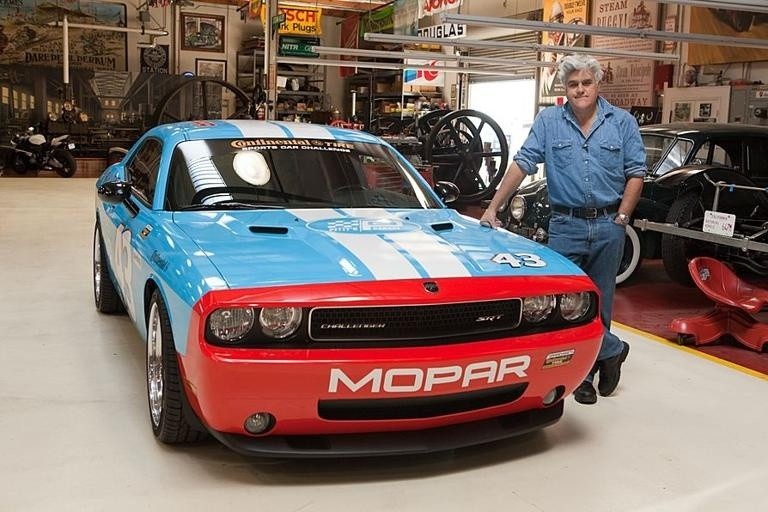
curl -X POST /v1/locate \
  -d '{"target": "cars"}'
[503,117,766,295]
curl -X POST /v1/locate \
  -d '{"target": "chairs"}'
[668,256,768,354]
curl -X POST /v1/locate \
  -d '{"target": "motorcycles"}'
[11,127,78,177]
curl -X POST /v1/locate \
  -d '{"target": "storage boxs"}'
[73,158,107,178]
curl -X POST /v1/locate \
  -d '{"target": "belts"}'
[547,199,623,222]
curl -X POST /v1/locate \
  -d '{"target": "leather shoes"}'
[572,381,597,406]
[599,338,629,395]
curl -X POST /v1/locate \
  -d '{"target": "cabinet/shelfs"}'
[236,48,333,126]
[343,43,470,174]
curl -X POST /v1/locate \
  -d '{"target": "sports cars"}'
[90,117,604,467]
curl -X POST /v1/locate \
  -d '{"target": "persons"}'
[480,53,647,405]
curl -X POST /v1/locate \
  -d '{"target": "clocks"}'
[140,43,169,75]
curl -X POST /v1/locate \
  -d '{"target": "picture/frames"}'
[536,0,592,106]
[0,0,128,72]
[195,58,227,83]
[180,12,225,53]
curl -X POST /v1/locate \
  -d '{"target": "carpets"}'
[460,200,768,381]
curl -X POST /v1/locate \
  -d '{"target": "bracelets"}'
[616,211,631,224]
